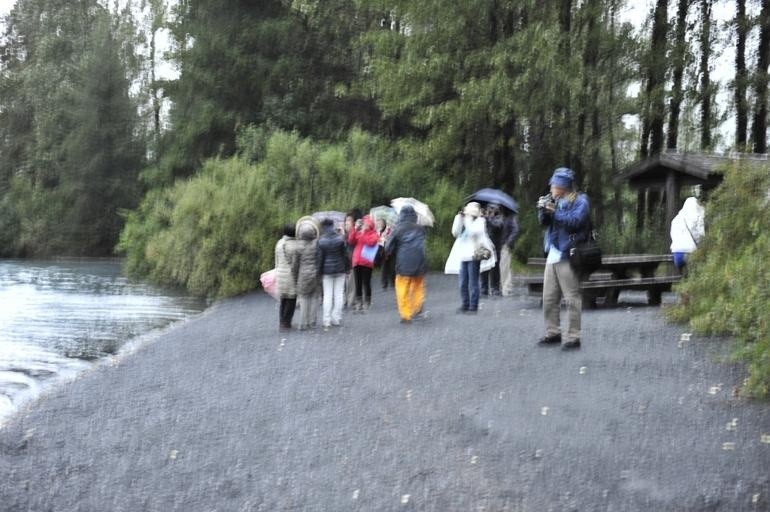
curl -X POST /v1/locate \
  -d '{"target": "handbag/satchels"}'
[570,246,602,273]
[473,246,491,261]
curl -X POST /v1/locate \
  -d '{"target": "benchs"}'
[578,274,683,310]
[522,273,638,309]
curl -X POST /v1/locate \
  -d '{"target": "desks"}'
[526,254,675,308]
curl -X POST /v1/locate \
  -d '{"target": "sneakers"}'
[564,342,581,349]
[539,334,561,344]
[481,287,501,297]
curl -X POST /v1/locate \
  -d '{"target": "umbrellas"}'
[389,197,436,228]
[466,189,520,211]
[260,269,277,300]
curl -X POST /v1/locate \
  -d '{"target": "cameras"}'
[538,196,555,208]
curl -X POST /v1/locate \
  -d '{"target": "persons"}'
[482,199,505,297]
[274,204,428,330]
[500,214,518,296]
[537,168,591,350]
[669,198,704,273]
[444,198,496,314]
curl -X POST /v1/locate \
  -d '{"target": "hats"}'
[362,218,374,229]
[551,168,575,188]
[463,201,481,218]
